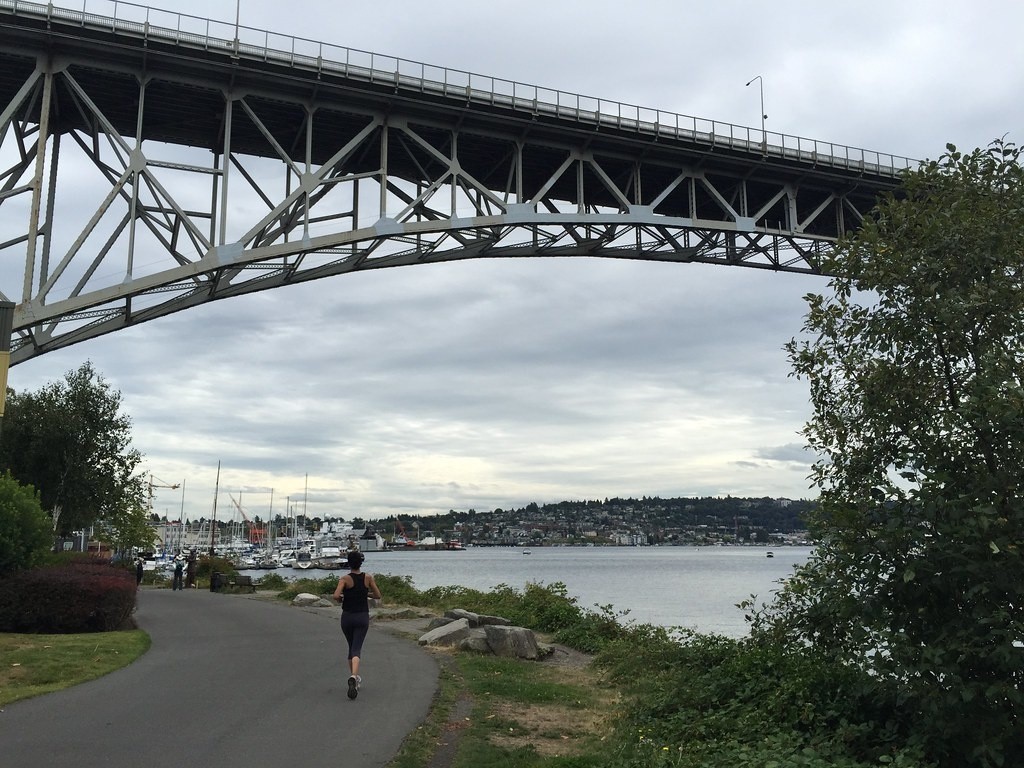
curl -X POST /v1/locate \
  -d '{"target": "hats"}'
[176,554,183,560]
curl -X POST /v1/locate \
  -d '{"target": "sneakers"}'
[347,675,361,699]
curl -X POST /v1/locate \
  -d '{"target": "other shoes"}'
[180,589,183,591]
[136,589,141,593]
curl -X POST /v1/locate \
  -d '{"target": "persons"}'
[134,558,144,591]
[333,550,380,699]
[172,554,185,591]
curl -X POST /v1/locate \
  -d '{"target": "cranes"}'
[145,474,181,521]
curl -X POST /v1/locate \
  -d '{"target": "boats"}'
[766,551,773,557]
[375,533,470,553]
[131,459,366,570]
[522,547,532,554]
